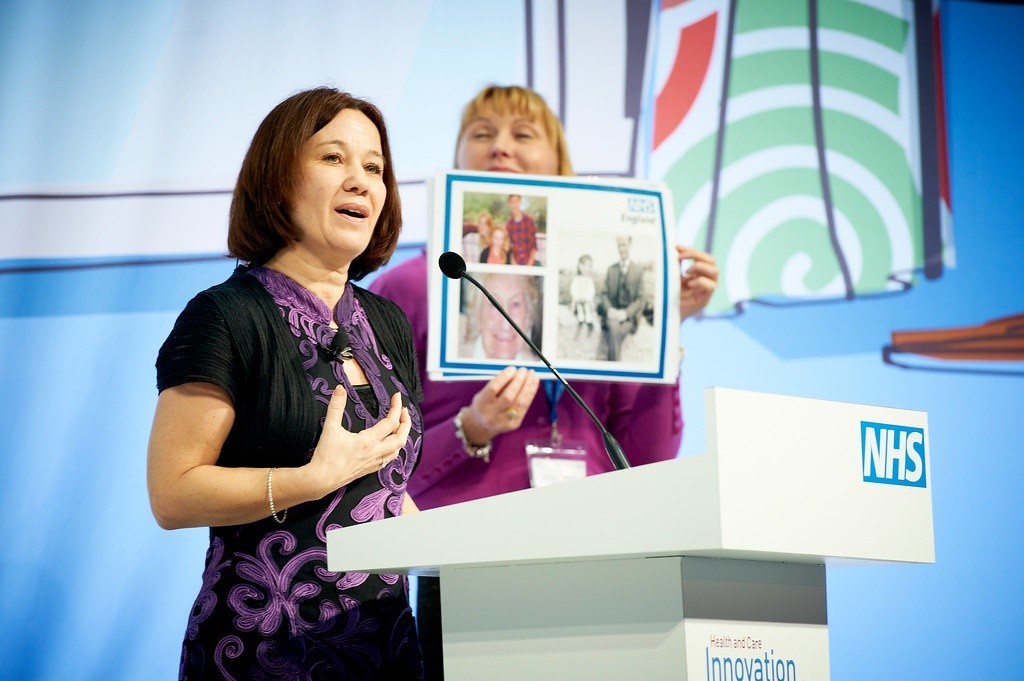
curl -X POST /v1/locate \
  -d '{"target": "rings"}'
[378,457,384,467]
[506,408,519,420]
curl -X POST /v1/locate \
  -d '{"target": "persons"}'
[463,195,538,265]
[369,84,720,510]
[571,254,596,325]
[597,235,648,362]
[147,86,425,681]
[459,274,542,362]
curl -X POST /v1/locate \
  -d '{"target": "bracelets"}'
[454,407,491,463]
[268,468,287,523]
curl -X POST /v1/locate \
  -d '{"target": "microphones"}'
[439,252,630,470]
